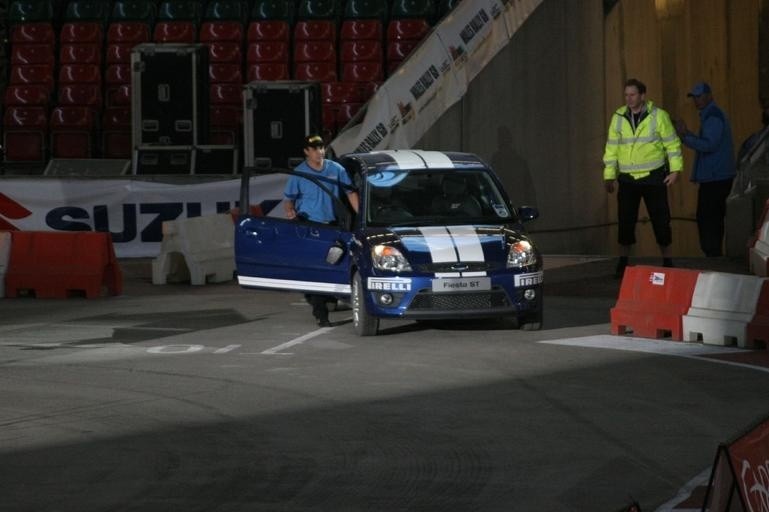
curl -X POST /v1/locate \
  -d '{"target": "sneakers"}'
[617,256,628,279]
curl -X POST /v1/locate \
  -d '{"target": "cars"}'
[229,145,547,334]
[723,127,769,278]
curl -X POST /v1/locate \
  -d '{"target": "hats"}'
[304,134,324,146]
[687,82,711,97]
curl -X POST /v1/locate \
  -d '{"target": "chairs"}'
[367,171,484,225]
[0,1,454,172]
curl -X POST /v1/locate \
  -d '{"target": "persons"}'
[603,79,684,279]
[676,82,737,256]
[282,136,359,328]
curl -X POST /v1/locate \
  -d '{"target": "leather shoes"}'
[319,320,332,327]
